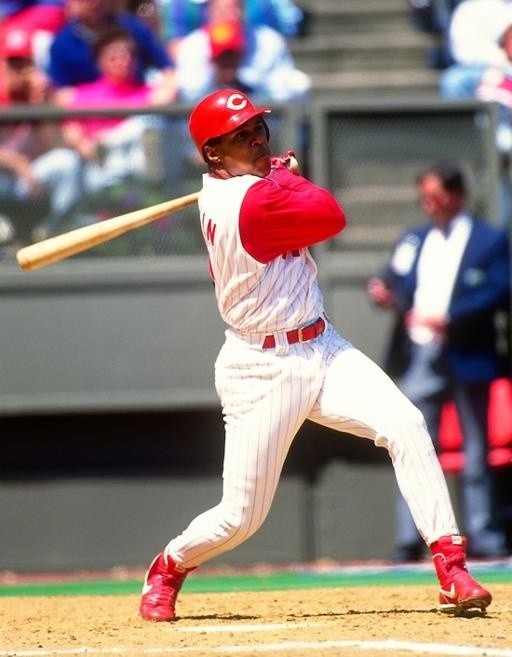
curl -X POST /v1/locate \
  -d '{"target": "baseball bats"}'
[16,154,301,270]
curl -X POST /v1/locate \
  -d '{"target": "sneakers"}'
[428,536,492,611]
[139,550,197,622]
[386,543,426,561]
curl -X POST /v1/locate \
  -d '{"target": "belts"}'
[263,313,330,351]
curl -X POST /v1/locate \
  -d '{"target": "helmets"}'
[187,87,272,162]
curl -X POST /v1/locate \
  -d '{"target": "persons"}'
[412,1,511,156]
[0,0,308,248]
[138,87,493,623]
[361,156,511,561]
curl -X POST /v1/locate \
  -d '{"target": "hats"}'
[1,24,33,61]
[205,16,246,58]
